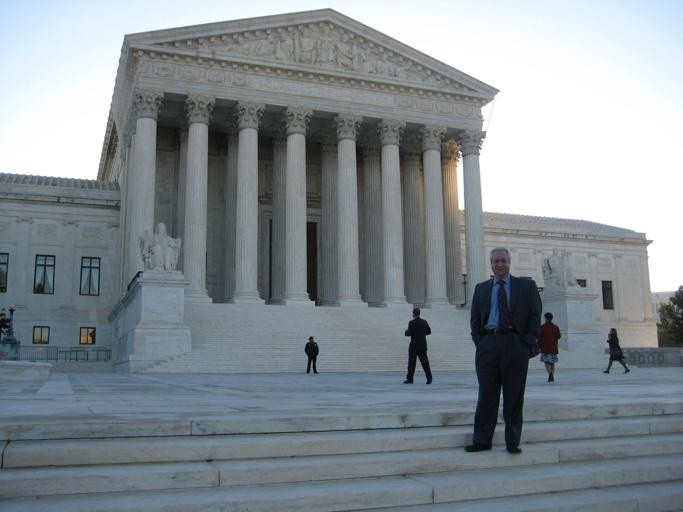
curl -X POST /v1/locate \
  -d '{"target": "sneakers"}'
[547,373,554,383]
[603,370,609,374]
[403,378,415,384]
[624,369,630,374]
[426,379,434,384]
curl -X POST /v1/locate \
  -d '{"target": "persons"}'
[464,247,542,453]
[305,336,319,374]
[404,307,433,384]
[602,328,630,374]
[536,312,561,382]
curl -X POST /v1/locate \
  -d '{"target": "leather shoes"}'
[464,441,492,452]
[507,445,522,453]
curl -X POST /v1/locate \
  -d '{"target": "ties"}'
[496,280,509,331]
